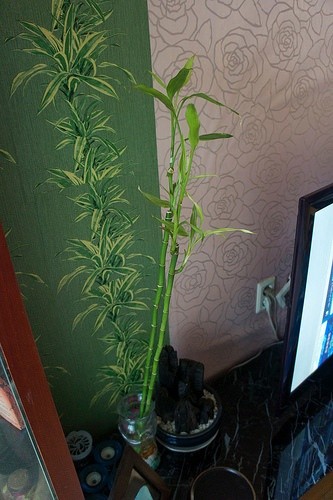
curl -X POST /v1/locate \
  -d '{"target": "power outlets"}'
[255,275,277,315]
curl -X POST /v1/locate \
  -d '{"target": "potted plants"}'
[5,1,258,473]
[149,344,224,473]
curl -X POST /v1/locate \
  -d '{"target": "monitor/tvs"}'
[279,183,332,413]
